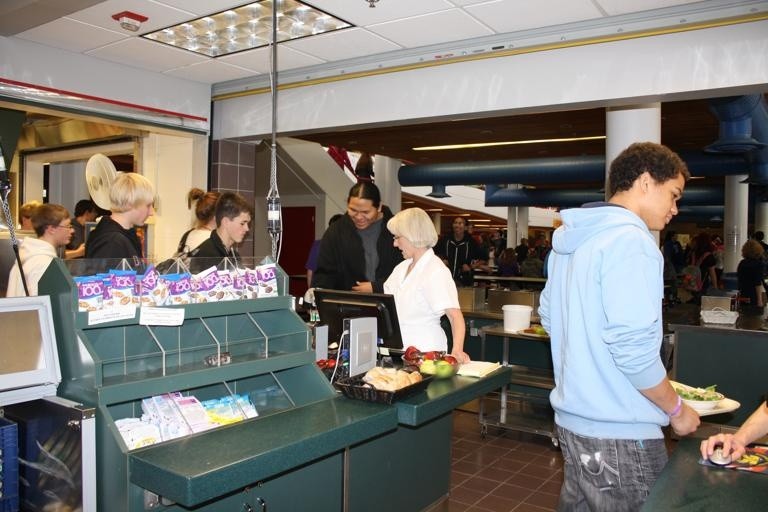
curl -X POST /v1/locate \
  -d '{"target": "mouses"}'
[709,447,734,466]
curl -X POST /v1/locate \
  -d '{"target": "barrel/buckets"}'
[501,304,533,336]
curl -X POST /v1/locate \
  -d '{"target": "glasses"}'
[51,223,73,229]
[393,235,401,241]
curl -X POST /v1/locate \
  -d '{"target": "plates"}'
[669,380,741,417]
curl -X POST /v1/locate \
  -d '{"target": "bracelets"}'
[666,396,684,418]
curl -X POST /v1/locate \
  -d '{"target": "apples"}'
[405,346,420,361]
[440,356,458,375]
[433,360,454,380]
[422,351,442,361]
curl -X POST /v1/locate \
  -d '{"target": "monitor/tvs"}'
[313,288,405,350]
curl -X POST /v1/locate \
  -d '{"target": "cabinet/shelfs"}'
[479,328,561,450]
[38,256,511,512]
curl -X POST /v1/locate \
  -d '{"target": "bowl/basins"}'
[401,354,464,379]
[678,389,725,410]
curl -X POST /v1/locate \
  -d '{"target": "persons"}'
[701,396,767,462]
[6,204,74,297]
[17,202,38,234]
[178,188,218,255]
[470,229,551,291]
[191,193,252,273]
[86,173,157,273]
[735,242,764,313]
[381,207,469,363]
[303,182,404,323]
[63,199,96,260]
[538,141,700,511]
[441,217,476,286]
[753,232,768,280]
[660,229,727,305]
[306,213,345,324]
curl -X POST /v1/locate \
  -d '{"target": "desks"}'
[639,420,768,511]
